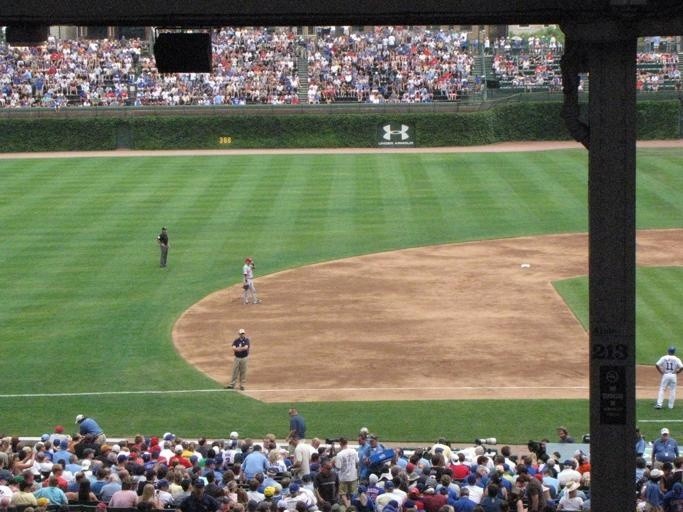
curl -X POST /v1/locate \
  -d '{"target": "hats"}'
[239,329,245,335]
[246,258,251,261]
[75,415,84,424]
[366,433,377,441]
[38,426,326,509]
[385,480,394,490]
[370,473,378,484]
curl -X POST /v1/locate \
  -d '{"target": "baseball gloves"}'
[242,283,249,291]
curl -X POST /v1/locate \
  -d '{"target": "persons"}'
[653,346,683,409]
[286,408,306,457]
[240,257,263,304]
[224,328,250,391]
[1,425,683,512]
[0,23,683,108]
[157,226,169,268]
[75,414,106,445]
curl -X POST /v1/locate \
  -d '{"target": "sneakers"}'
[391,465,401,475]
